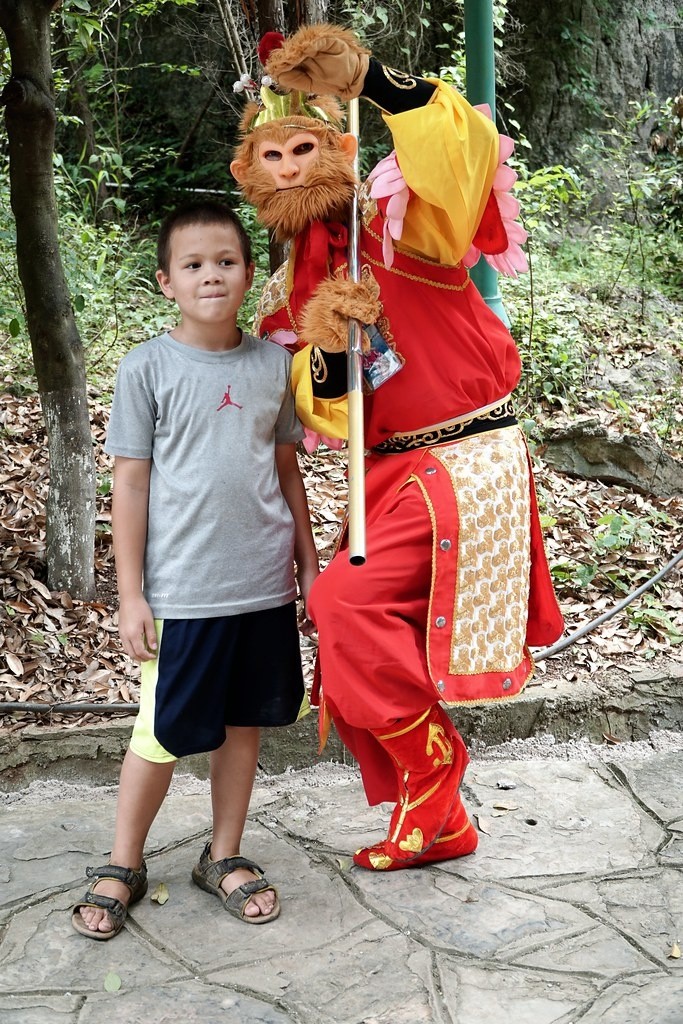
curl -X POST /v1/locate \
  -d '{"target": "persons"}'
[228,21,565,871]
[71,206,322,940]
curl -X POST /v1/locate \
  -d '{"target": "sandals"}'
[191,842,282,923]
[74,857,148,937]
